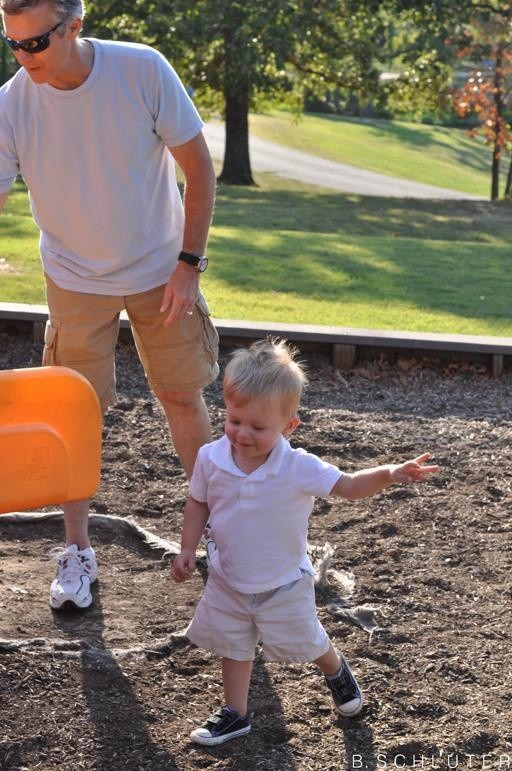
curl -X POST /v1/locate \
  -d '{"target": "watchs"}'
[177,252,210,273]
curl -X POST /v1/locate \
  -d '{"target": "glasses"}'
[2,18,67,53]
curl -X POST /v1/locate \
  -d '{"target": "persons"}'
[172,335,441,746]
[0,0,219,613]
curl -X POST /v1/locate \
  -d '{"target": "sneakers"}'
[190,706,252,746]
[324,652,364,717]
[49,543,99,613]
